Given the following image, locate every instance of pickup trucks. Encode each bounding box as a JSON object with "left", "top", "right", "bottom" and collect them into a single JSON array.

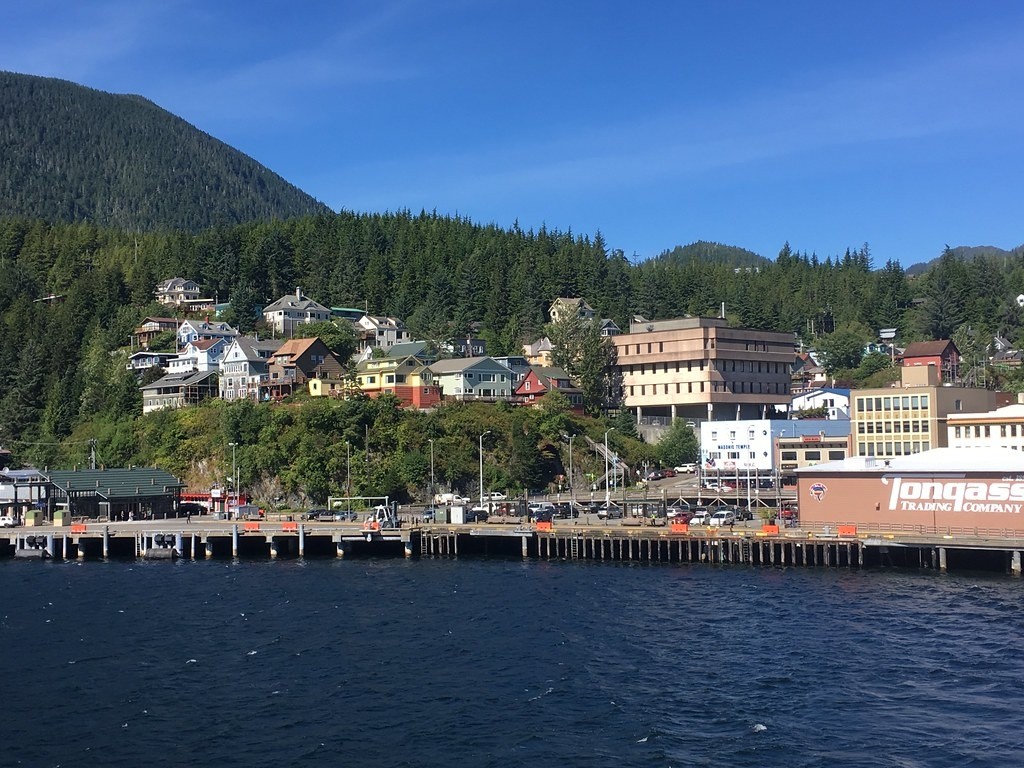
[
  {"left": 553, "top": 505, "right": 579, "bottom": 519},
  {"left": 580, "top": 503, "right": 600, "bottom": 514},
  {"left": 480, "top": 492, "right": 508, "bottom": 501}
]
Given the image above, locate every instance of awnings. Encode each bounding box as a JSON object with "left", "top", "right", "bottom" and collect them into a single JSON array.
[{"left": 778, "top": 500, "right": 798, "bottom": 524}]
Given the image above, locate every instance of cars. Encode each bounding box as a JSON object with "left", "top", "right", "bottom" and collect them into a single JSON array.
[
  {"left": 597, "top": 506, "right": 623, "bottom": 520},
  {"left": 778, "top": 507, "right": 798, "bottom": 520},
  {"left": 177, "top": 502, "right": 207, "bottom": 517},
  {"left": 647, "top": 469, "right": 676, "bottom": 483},
  {"left": 314, "top": 510, "right": 340, "bottom": 521},
  {"left": 674, "top": 464, "right": 698, "bottom": 474},
  {"left": 335, "top": 511, "right": 358, "bottom": 520},
  {"left": 690, "top": 514, "right": 711, "bottom": 526},
  {"left": 300, "top": 509, "right": 325, "bottom": 521},
  {"left": 421, "top": 509, "right": 435, "bottom": 520},
  {"left": 731, "top": 507, "right": 753, "bottom": 520},
  {"left": 632, "top": 504, "right": 733, "bottom": 519},
  {"left": 0, "top": 516, "right": 18, "bottom": 528},
  {"left": 710, "top": 511, "right": 736, "bottom": 527},
  {"left": 651, "top": 420, "right": 661, "bottom": 426},
  {"left": 686, "top": 422, "right": 696, "bottom": 427},
  {"left": 672, "top": 512, "right": 692, "bottom": 525},
  {"left": 706, "top": 483, "right": 732, "bottom": 493},
  {"left": 528, "top": 509, "right": 553, "bottom": 523},
  {"left": 465, "top": 502, "right": 525, "bottom": 522}
]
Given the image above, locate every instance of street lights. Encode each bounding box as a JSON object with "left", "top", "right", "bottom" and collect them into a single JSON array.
[
  {"left": 429, "top": 439, "right": 434, "bottom": 510},
  {"left": 345, "top": 441, "right": 351, "bottom": 521},
  {"left": 479, "top": 431, "right": 491, "bottom": 510},
  {"left": 563, "top": 433, "right": 576, "bottom": 523},
  {"left": 229, "top": 442, "right": 238, "bottom": 508},
  {"left": 604, "top": 427, "right": 615, "bottom": 508}
]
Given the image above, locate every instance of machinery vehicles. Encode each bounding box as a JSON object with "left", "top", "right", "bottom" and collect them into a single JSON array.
[{"left": 363, "top": 500, "right": 401, "bottom": 530}]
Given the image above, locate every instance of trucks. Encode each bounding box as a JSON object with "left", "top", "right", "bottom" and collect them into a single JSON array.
[
  {"left": 435, "top": 493, "right": 463, "bottom": 505},
  {"left": 528, "top": 502, "right": 553, "bottom": 513}
]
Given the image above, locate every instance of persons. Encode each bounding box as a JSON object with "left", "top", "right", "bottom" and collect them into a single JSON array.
[
  {"left": 20, "top": 514, "right": 25, "bottom": 527},
  {"left": 769, "top": 516, "right": 775, "bottom": 525},
  {"left": 650, "top": 513, "right": 656, "bottom": 526},
  {"left": 121, "top": 511, "right": 125, "bottom": 521},
  {"left": 260, "top": 514, "right": 265, "bottom": 518},
  {"left": 143, "top": 511, "right": 147, "bottom": 519},
  {"left": 187, "top": 512, "right": 191, "bottom": 525},
  {"left": 730, "top": 525, "right": 735, "bottom": 532}
]
